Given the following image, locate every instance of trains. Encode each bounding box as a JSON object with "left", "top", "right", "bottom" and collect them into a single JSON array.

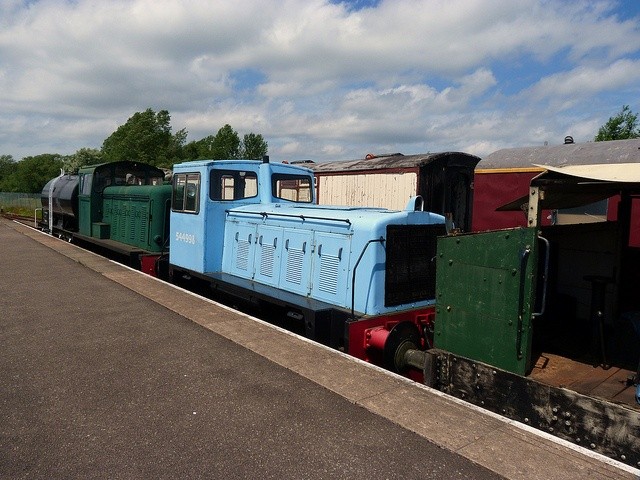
[
  {"left": 125, "top": 135, "right": 640, "bottom": 246},
  {"left": 32, "top": 155, "right": 640, "bottom": 470}
]
